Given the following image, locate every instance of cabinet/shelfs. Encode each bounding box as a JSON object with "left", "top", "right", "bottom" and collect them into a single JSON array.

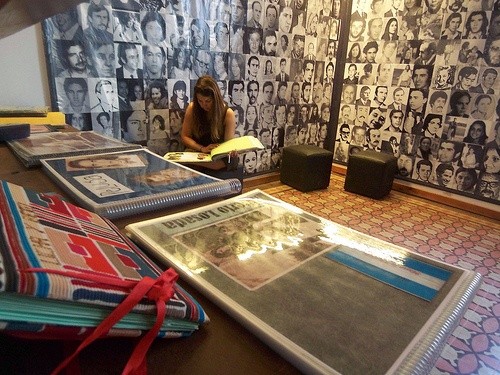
[{"left": 0, "top": 0, "right": 302, "bottom": 375}]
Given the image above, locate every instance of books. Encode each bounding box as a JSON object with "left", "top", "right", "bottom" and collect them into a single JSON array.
[
  {"left": 124, "top": 188, "right": 482, "bottom": 375},
  {"left": 0, "top": 110, "right": 143, "bottom": 169},
  {"left": 162, "top": 135, "right": 265, "bottom": 165},
  {"left": 39, "top": 148, "right": 243, "bottom": 220}
]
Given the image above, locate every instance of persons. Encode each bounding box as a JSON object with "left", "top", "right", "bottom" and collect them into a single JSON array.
[
  {"left": 43, "top": 0, "right": 342, "bottom": 177},
  {"left": 68, "top": 155, "right": 141, "bottom": 169},
  {"left": 176, "top": 76, "right": 240, "bottom": 171},
  {"left": 333, "top": 0, "right": 500, "bottom": 202},
  {"left": 212, "top": 211, "right": 305, "bottom": 258}
]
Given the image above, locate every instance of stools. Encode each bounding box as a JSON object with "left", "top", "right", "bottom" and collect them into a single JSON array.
[
  {"left": 279, "top": 144, "right": 333, "bottom": 192},
  {"left": 343, "top": 149, "right": 399, "bottom": 201}
]
